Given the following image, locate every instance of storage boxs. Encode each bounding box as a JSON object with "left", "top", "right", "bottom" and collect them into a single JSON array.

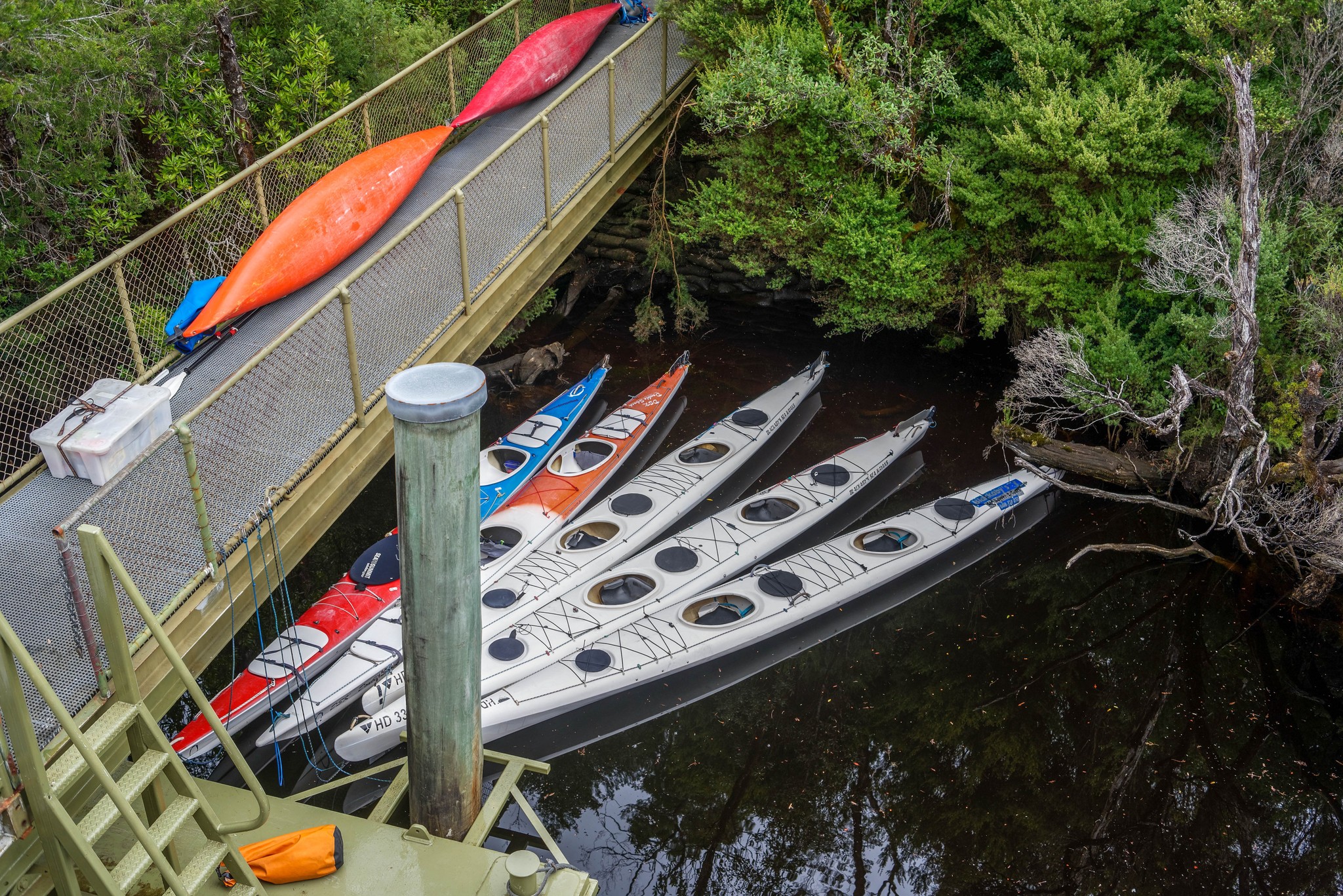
[{"left": 29, "top": 378, "right": 173, "bottom": 487}]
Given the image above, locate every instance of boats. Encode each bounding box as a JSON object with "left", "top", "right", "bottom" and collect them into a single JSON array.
[
  {"left": 483, "top": 493, "right": 1057, "bottom": 783},
  {"left": 342, "top": 453, "right": 927, "bottom": 819},
  {"left": 169, "top": 351, "right": 612, "bottom": 762},
  {"left": 210, "top": 397, "right": 688, "bottom": 788},
  {"left": 405, "top": 461, "right": 1064, "bottom": 761},
  {"left": 450, "top": 0, "right": 627, "bottom": 155},
  {"left": 254, "top": 351, "right": 694, "bottom": 749},
  {"left": 164, "top": 126, "right": 452, "bottom": 355},
  {"left": 328, "top": 403, "right": 941, "bottom": 766},
  {"left": 290, "top": 392, "right": 828, "bottom": 804},
  {"left": 355, "top": 351, "right": 834, "bottom": 718}
]
